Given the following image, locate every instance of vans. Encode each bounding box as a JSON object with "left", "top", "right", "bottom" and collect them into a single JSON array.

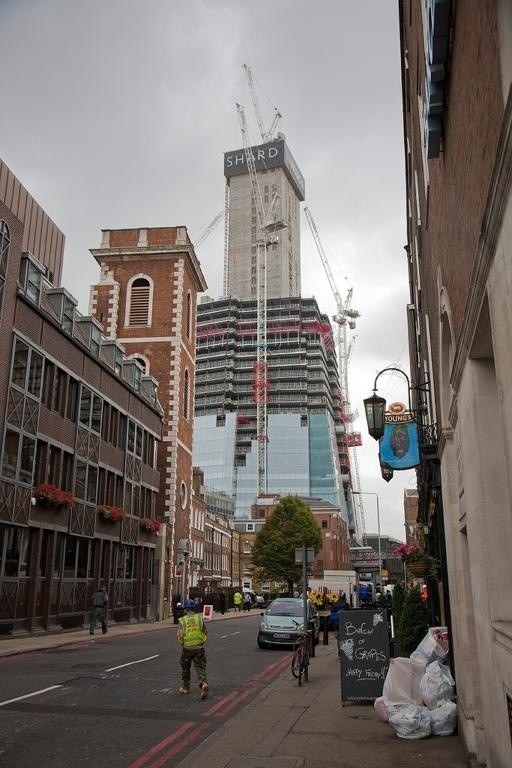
[{"left": 256, "top": 597, "right": 321, "bottom": 650}]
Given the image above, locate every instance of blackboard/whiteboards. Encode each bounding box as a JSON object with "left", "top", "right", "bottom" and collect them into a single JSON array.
[{"left": 338, "top": 610, "right": 390, "bottom": 702}]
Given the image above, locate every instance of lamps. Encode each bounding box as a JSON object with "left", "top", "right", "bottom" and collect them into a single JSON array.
[
  {"left": 381, "top": 462, "right": 395, "bottom": 483},
  {"left": 365, "top": 365, "right": 446, "bottom": 447}
]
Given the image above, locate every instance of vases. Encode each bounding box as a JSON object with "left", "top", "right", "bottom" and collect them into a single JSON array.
[
  {"left": 97, "top": 511, "right": 113, "bottom": 524},
  {"left": 406, "top": 560, "right": 428, "bottom": 577},
  {"left": 140, "top": 525, "right": 150, "bottom": 534},
  {"left": 38, "top": 496, "right": 67, "bottom": 512}
]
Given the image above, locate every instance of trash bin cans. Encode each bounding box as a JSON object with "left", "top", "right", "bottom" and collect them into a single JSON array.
[{"left": 173, "top": 606, "right": 184, "bottom": 624}]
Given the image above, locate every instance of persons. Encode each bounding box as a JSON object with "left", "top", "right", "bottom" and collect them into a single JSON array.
[
  {"left": 176, "top": 598, "right": 209, "bottom": 700},
  {"left": 339, "top": 588, "right": 347, "bottom": 601},
  {"left": 233, "top": 590, "right": 265, "bottom": 613},
  {"left": 330, "top": 597, "right": 350, "bottom": 658},
  {"left": 219, "top": 590, "right": 225, "bottom": 617},
  {"left": 375, "top": 587, "right": 394, "bottom": 610},
  {"left": 88, "top": 585, "right": 108, "bottom": 636},
  {"left": 293, "top": 588, "right": 304, "bottom": 598}
]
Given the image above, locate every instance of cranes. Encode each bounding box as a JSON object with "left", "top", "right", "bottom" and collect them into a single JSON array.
[
  {"left": 234, "top": 102, "right": 290, "bottom": 496},
  {"left": 302, "top": 205, "right": 366, "bottom": 542},
  {"left": 189, "top": 209, "right": 226, "bottom": 252},
  {"left": 241, "top": 61, "right": 282, "bottom": 142}
]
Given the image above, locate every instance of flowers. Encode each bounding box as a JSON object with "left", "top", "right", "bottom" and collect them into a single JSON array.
[
  {"left": 32, "top": 482, "right": 74, "bottom": 507},
  {"left": 96, "top": 502, "right": 125, "bottom": 522},
  {"left": 139, "top": 516, "right": 161, "bottom": 534},
  {"left": 392, "top": 542, "right": 427, "bottom": 562}
]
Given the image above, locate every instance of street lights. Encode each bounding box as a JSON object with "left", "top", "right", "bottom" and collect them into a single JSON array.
[{"left": 351, "top": 491, "right": 383, "bottom": 595}]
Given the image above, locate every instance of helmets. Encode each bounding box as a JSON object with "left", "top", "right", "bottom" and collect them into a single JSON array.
[{"left": 183, "top": 599, "right": 196, "bottom": 608}]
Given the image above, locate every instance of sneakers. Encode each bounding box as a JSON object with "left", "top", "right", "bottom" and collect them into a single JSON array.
[
  {"left": 179, "top": 687, "right": 188, "bottom": 693},
  {"left": 201, "top": 683, "right": 209, "bottom": 699}
]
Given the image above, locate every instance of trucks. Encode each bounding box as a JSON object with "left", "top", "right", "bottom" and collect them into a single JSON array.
[{"left": 243, "top": 587, "right": 265, "bottom": 606}]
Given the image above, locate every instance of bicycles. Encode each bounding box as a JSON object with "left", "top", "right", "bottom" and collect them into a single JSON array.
[{"left": 289, "top": 619, "right": 313, "bottom": 687}]
